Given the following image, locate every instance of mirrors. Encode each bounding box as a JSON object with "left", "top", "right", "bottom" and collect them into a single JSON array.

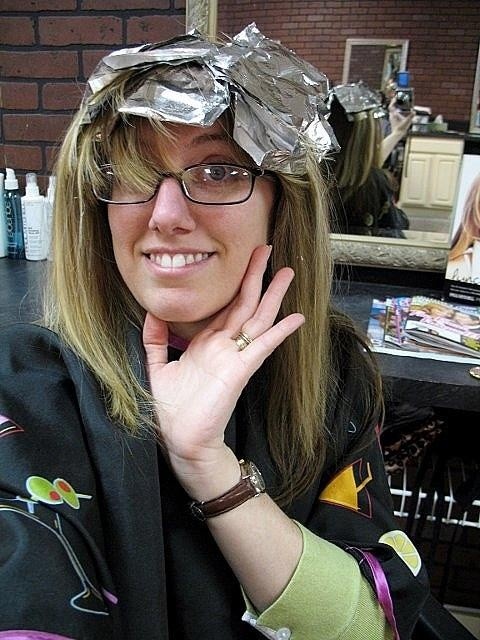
[
  {"left": 186, "top": 0, "right": 480, "bottom": 275},
  {"left": 342, "top": 38, "right": 410, "bottom": 97},
  {"left": 468, "top": 43, "right": 480, "bottom": 134},
  {"left": 381, "top": 47, "right": 403, "bottom": 94}
]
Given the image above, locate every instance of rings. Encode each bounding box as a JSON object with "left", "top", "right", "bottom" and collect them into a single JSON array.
[{"left": 231, "top": 332, "right": 253, "bottom": 351}]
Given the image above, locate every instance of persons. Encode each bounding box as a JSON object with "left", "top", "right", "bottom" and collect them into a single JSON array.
[
  {"left": 0, "top": 21, "right": 433, "bottom": 639},
  {"left": 323, "top": 81, "right": 417, "bottom": 230}
]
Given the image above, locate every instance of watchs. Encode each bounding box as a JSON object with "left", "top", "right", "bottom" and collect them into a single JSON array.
[{"left": 187, "top": 459, "right": 267, "bottom": 523}]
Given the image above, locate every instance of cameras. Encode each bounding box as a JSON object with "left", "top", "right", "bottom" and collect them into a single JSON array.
[{"left": 396, "top": 87, "right": 415, "bottom": 114}]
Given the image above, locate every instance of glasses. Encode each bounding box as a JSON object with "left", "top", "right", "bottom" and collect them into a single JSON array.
[{"left": 86, "top": 158, "right": 276, "bottom": 209}]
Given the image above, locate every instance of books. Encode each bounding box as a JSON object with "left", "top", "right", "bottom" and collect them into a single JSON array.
[
  {"left": 441, "top": 154, "right": 480, "bottom": 305},
  {"left": 361, "top": 293, "right": 480, "bottom": 368}
]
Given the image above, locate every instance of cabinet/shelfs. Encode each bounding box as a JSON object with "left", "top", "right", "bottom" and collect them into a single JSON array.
[{"left": 400, "top": 136, "right": 465, "bottom": 209}]
